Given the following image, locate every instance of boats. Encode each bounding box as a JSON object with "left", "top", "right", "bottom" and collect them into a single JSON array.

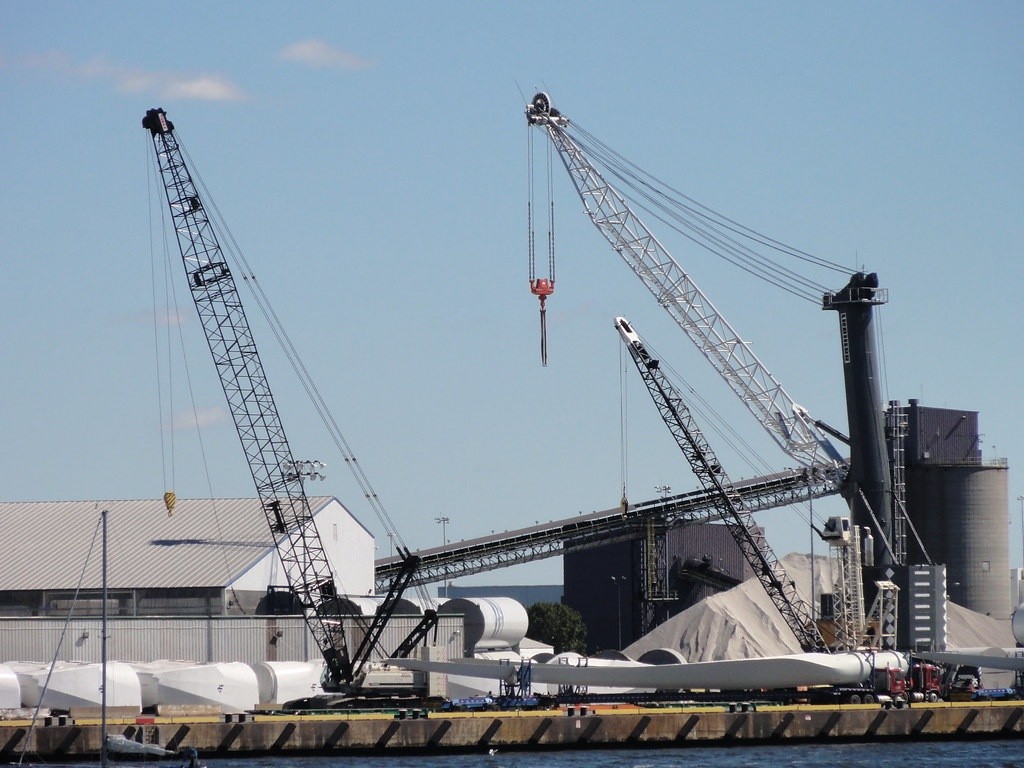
[{"left": 105, "top": 732, "right": 177, "bottom": 761}]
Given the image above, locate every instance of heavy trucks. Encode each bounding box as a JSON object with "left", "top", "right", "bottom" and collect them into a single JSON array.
[{"left": 811, "top": 662, "right": 943, "bottom": 704}]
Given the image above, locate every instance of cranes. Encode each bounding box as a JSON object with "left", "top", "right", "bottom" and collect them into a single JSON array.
[
  {"left": 521, "top": 90, "right": 949, "bottom": 704},
  {"left": 140, "top": 105, "right": 454, "bottom": 714}
]
